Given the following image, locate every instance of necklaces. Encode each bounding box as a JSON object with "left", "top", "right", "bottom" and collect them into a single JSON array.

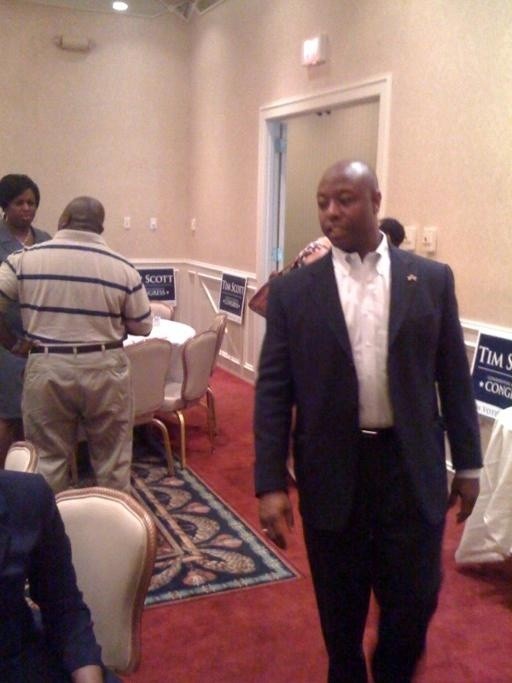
[{"left": 22, "top": 228, "right": 32, "bottom": 246}]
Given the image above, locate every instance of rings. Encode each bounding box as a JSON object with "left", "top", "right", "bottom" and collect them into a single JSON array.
[{"left": 262, "top": 529, "right": 268, "bottom": 533}]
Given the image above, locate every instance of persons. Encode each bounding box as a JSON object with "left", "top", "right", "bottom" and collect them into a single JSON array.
[
  {"left": 251, "top": 159, "right": 484, "bottom": 683},
  {"left": 0, "top": 471, "right": 125, "bottom": 682},
  {"left": 0, "top": 197, "right": 153, "bottom": 498},
  {"left": 0, "top": 174, "right": 53, "bottom": 470},
  {"left": 379, "top": 219, "right": 405, "bottom": 248}
]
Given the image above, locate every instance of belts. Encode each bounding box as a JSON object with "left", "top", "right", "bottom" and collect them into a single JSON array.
[
  {"left": 30, "top": 342, "right": 123, "bottom": 354},
  {"left": 360, "top": 425, "right": 396, "bottom": 445}
]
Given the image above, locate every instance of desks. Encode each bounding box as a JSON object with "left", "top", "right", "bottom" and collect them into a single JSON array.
[{"left": 126, "top": 321, "right": 194, "bottom": 361}]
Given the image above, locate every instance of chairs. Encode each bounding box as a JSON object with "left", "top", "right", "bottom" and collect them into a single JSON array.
[
  {"left": 123, "top": 338, "right": 179, "bottom": 481},
  {"left": 55, "top": 486, "right": 159, "bottom": 676},
  {"left": 148, "top": 300, "right": 177, "bottom": 321},
  {"left": 160, "top": 328, "right": 218, "bottom": 469},
  {"left": 1, "top": 442, "right": 35, "bottom": 476}
]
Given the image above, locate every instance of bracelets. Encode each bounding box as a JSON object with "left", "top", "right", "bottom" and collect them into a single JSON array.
[{"left": 10, "top": 339, "right": 22, "bottom": 354}]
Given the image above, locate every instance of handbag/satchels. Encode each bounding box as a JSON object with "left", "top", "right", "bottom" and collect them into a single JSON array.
[{"left": 248, "top": 257, "right": 297, "bottom": 318}]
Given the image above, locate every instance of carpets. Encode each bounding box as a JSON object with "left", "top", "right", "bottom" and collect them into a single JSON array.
[{"left": 77, "top": 435, "right": 303, "bottom": 608}]
